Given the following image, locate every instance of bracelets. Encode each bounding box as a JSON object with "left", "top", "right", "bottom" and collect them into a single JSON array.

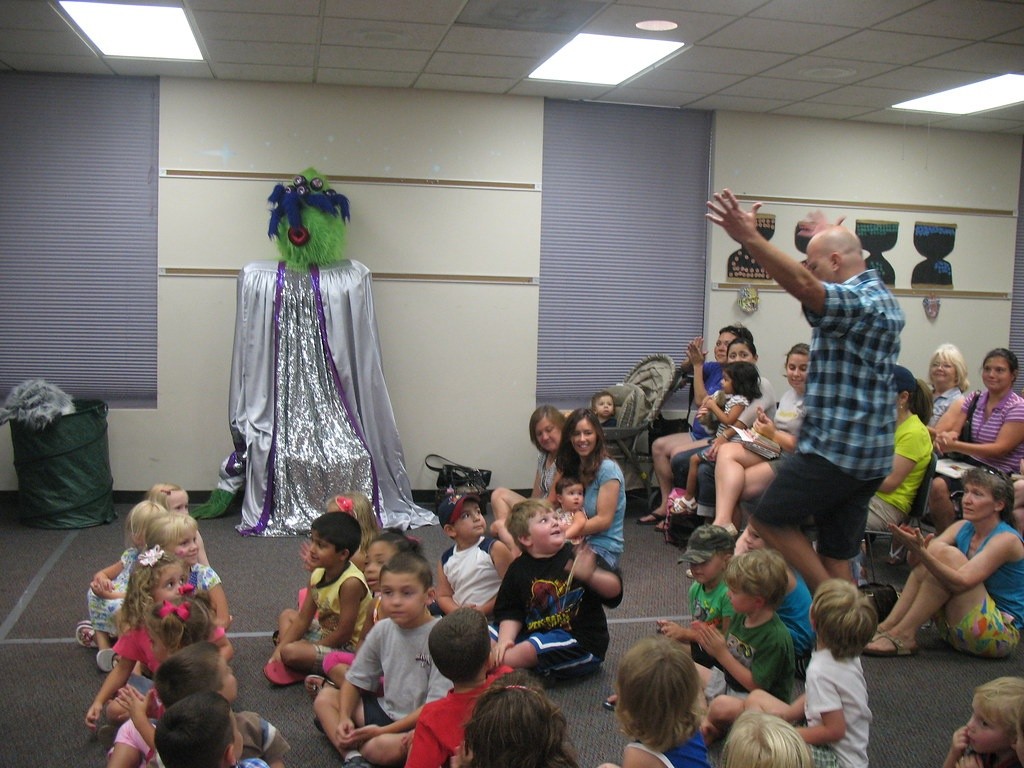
[{"left": 722, "top": 432, "right": 730, "bottom": 441}]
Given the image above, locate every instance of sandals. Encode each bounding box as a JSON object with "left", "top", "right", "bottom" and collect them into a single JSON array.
[
  {"left": 654, "top": 520, "right": 670, "bottom": 533},
  {"left": 76, "top": 624, "right": 103, "bottom": 649},
  {"left": 672, "top": 496, "right": 697, "bottom": 514},
  {"left": 635, "top": 511, "right": 667, "bottom": 524},
  {"left": 303, "top": 673, "right": 338, "bottom": 693},
  {"left": 96, "top": 647, "right": 118, "bottom": 673},
  {"left": 888, "top": 540, "right": 908, "bottom": 566}
]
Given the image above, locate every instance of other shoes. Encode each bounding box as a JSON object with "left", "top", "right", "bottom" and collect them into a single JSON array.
[
  {"left": 339, "top": 756, "right": 377, "bottom": 767},
  {"left": 264, "top": 658, "right": 305, "bottom": 688},
  {"left": 723, "top": 523, "right": 740, "bottom": 536}
]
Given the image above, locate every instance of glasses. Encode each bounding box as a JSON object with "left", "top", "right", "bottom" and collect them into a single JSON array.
[{"left": 980, "top": 464, "right": 1002, "bottom": 478}]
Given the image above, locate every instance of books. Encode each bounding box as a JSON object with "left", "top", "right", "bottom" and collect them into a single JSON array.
[{"left": 742, "top": 427, "right": 781, "bottom": 459}]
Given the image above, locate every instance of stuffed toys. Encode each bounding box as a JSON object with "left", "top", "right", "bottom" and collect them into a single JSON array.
[{"left": 190, "top": 165, "right": 440, "bottom": 538}]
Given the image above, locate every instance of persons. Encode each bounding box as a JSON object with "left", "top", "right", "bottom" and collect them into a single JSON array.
[
  {"left": 488, "top": 498, "right": 623, "bottom": 677},
  {"left": 926, "top": 342, "right": 1024, "bottom": 543},
  {"left": 637, "top": 325, "right": 777, "bottom": 547},
  {"left": 863, "top": 468, "right": 1024, "bottom": 657},
  {"left": 450, "top": 669, "right": 580, "bottom": 768},
  {"left": 598, "top": 634, "right": 712, "bottom": 768},
  {"left": 744, "top": 577, "right": 878, "bottom": 768},
  {"left": 427, "top": 494, "right": 513, "bottom": 621},
  {"left": 263, "top": 492, "right": 423, "bottom": 697},
  {"left": 943, "top": 677, "right": 1024, "bottom": 768},
  {"left": 564, "top": 391, "right": 617, "bottom": 427},
  {"left": 75, "top": 483, "right": 291, "bottom": 768},
  {"left": 490, "top": 404, "right": 626, "bottom": 570},
  {"left": 602, "top": 521, "right": 815, "bottom": 748},
  {"left": 718, "top": 712, "right": 816, "bottom": 768},
  {"left": 864, "top": 365, "right": 934, "bottom": 531},
  {"left": 404, "top": 606, "right": 514, "bottom": 768},
  {"left": 705, "top": 189, "right": 906, "bottom": 600},
  {"left": 686, "top": 344, "right": 811, "bottom": 578},
  {"left": 313, "top": 552, "right": 454, "bottom": 768}
]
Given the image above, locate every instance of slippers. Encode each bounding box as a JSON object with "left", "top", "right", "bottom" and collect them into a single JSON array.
[{"left": 864, "top": 627, "right": 919, "bottom": 656}]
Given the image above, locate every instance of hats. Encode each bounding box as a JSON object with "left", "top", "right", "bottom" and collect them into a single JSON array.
[
  {"left": 437, "top": 491, "right": 483, "bottom": 527},
  {"left": 677, "top": 524, "right": 735, "bottom": 559}
]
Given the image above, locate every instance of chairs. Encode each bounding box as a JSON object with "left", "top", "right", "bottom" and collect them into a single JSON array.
[{"left": 866, "top": 454, "right": 943, "bottom": 586}]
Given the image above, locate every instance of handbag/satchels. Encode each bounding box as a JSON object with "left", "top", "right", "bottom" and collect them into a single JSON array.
[
  {"left": 649, "top": 416, "right": 693, "bottom": 451},
  {"left": 424, "top": 453, "right": 493, "bottom": 516},
  {"left": 862, "top": 581, "right": 899, "bottom": 624},
  {"left": 948, "top": 389, "right": 983, "bottom": 459}
]
